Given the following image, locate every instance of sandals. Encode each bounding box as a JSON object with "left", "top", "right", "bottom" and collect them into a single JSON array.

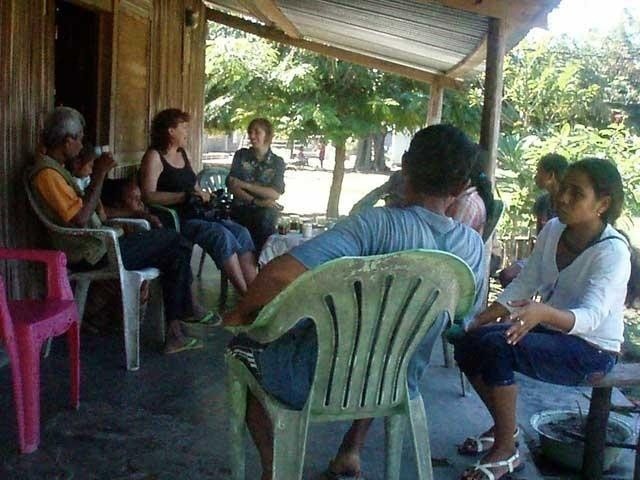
[
  {"left": 456, "top": 447, "right": 525, "bottom": 480},
  {"left": 457, "top": 425, "right": 520, "bottom": 454}
]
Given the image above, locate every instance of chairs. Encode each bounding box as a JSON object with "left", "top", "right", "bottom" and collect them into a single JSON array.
[{"left": 225, "top": 249, "right": 476, "bottom": 479}]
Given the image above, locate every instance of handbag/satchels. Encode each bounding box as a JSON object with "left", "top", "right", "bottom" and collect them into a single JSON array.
[{"left": 180, "top": 189, "right": 227, "bottom": 221}]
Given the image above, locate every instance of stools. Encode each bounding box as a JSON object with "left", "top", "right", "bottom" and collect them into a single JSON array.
[{"left": 568, "top": 363, "right": 640, "bottom": 479}]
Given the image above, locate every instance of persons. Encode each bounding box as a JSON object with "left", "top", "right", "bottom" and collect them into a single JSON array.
[
  {"left": 139, "top": 107, "right": 257, "bottom": 292},
  {"left": 436, "top": 144, "right": 494, "bottom": 240},
  {"left": 226, "top": 119, "right": 287, "bottom": 259},
  {"left": 222, "top": 122, "right": 490, "bottom": 480},
  {"left": 496, "top": 150, "right": 569, "bottom": 287},
  {"left": 28, "top": 103, "right": 223, "bottom": 358},
  {"left": 349, "top": 169, "right": 407, "bottom": 217},
  {"left": 453, "top": 156, "right": 640, "bottom": 480}
]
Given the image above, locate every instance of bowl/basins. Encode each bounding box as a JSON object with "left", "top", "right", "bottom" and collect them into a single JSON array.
[{"left": 530, "top": 408, "right": 635, "bottom": 473}]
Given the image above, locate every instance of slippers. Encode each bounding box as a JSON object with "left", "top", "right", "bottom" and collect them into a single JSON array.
[
  {"left": 163, "top": 336, "right": 206, "bottom": 353},
  {"left": 178, "top": 310, "right": 223, "bottom": 328}
]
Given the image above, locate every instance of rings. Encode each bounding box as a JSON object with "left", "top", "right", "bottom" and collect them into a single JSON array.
[{"left": 517, "top": 318, "right": 525, "bottom": 327}]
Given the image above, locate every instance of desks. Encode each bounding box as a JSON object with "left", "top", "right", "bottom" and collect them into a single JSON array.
[{"left": 261, "top": 230, "right": 320, "bottom": 263}]
